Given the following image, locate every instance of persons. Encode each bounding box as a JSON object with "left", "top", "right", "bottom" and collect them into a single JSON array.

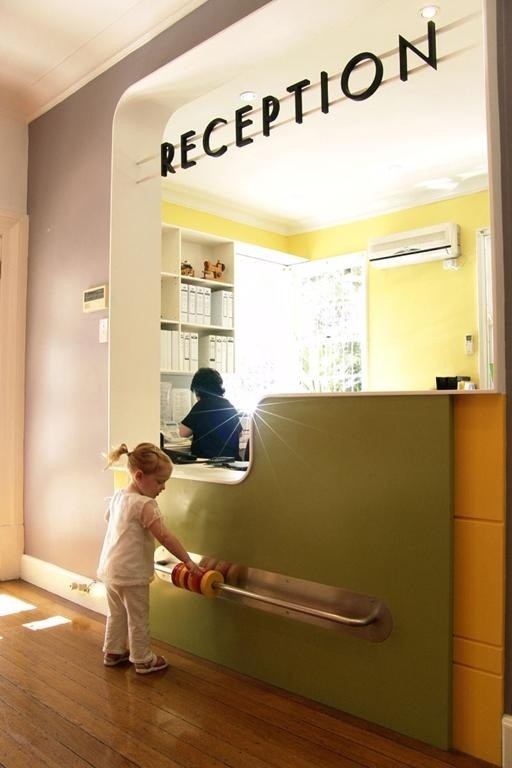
[
  {"left": 96, "top": 441, "right": 208, "bottom": 673},
  {"left": 178, "top": 367, "right": 244, "bottom": 463}
]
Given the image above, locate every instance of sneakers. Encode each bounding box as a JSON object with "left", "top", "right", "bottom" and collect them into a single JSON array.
[
  {"left": 134, "top": 653, "right": 168, "bottom": 674},
  {"left": 103, "top": 648, "right": 131, "bottom": 666}
]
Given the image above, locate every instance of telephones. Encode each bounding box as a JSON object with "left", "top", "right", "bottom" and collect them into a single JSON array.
[{"left": 464, "top": 334, "right": 475, "bottom": 356}]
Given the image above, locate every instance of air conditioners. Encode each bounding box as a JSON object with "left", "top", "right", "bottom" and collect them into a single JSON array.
[{"left": 366, "top": 223, "right": 462, "bottom": 270}]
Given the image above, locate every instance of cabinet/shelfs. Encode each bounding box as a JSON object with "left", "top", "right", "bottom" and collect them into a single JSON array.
[{"left": 162, "top": 223, "right": 237, "bottom": 430}]
